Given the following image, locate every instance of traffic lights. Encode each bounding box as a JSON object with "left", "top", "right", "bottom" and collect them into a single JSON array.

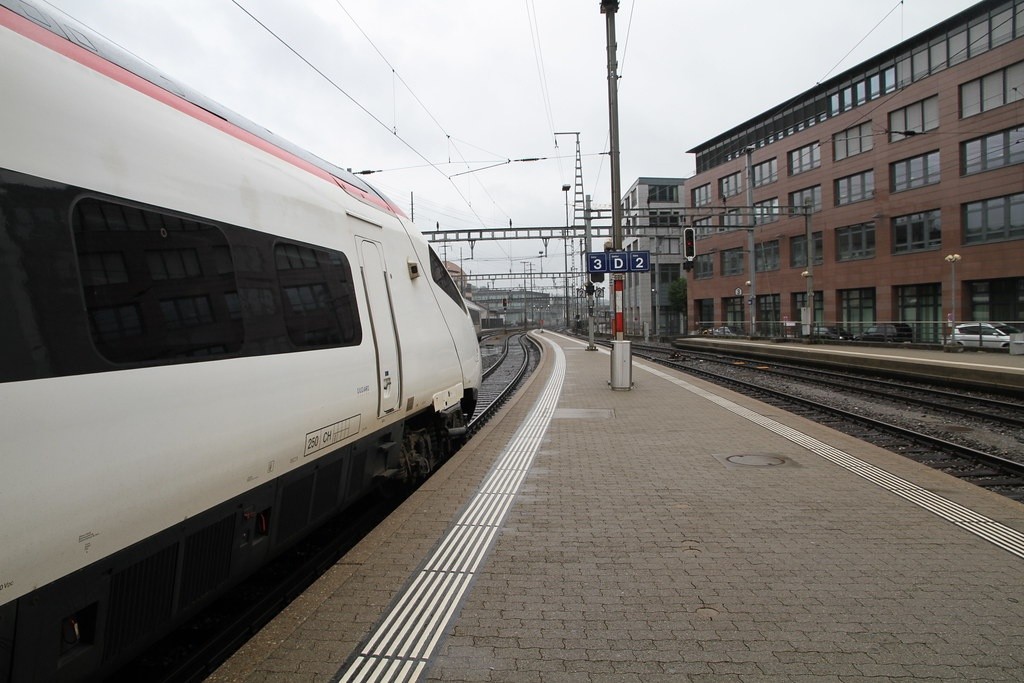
[
  {"left": 684, "top": 228, "right": 696, "bottom": 262},
  {"left": 503, "top": 299, "right": 507, "bottom": 307}
]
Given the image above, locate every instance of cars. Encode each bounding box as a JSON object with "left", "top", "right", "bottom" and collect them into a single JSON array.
[
  {"left": 951, "top": 321, "right": 1021, "bottom": 349},
  {"left": 714, "top": 325, "right": 747, "bottom": 336},
  {"left": 812, "top": 325, "right": 854, "bottom": 340},
  {"left": 854, "top": 322, "right": 913, "bottom": 343}
]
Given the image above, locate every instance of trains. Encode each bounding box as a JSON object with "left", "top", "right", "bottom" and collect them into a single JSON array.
[{"left": 1, "top": 1, "right": 481, "bottom": 682}]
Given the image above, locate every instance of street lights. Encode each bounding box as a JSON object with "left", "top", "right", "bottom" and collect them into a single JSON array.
[
  {"left": 562, "top": 183, "right": 571, "bottom": 235},
  {"left": 945, "top": 253, "right": 962, "bottom": 343},
  {"left": 744, "top": 145, "right": 757, "bottom": 339}
]
